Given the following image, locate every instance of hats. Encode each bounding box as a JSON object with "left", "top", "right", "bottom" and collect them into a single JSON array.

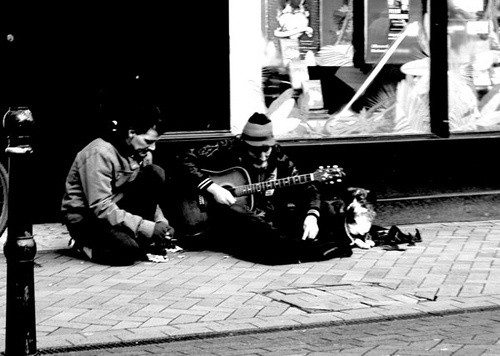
[{"left": 242, "top": 112, "right": 277, "bottom": 146}]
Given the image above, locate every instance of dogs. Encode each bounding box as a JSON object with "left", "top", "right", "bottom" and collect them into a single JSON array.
[{"left": 323, "top": 181, "right": 378, "bottom": 250}]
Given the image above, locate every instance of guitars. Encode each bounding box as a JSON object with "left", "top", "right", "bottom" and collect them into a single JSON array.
[{"left": 174, "top": 162, "right": 344, "bottom": 239}]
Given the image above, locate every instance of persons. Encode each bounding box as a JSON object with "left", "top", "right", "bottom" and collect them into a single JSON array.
[
  {"left": 61, "top": 103, "right": 176, "bottom": 267},
  {"left": 175, "top": 113, "right": 320, "bottom": 239}
]
